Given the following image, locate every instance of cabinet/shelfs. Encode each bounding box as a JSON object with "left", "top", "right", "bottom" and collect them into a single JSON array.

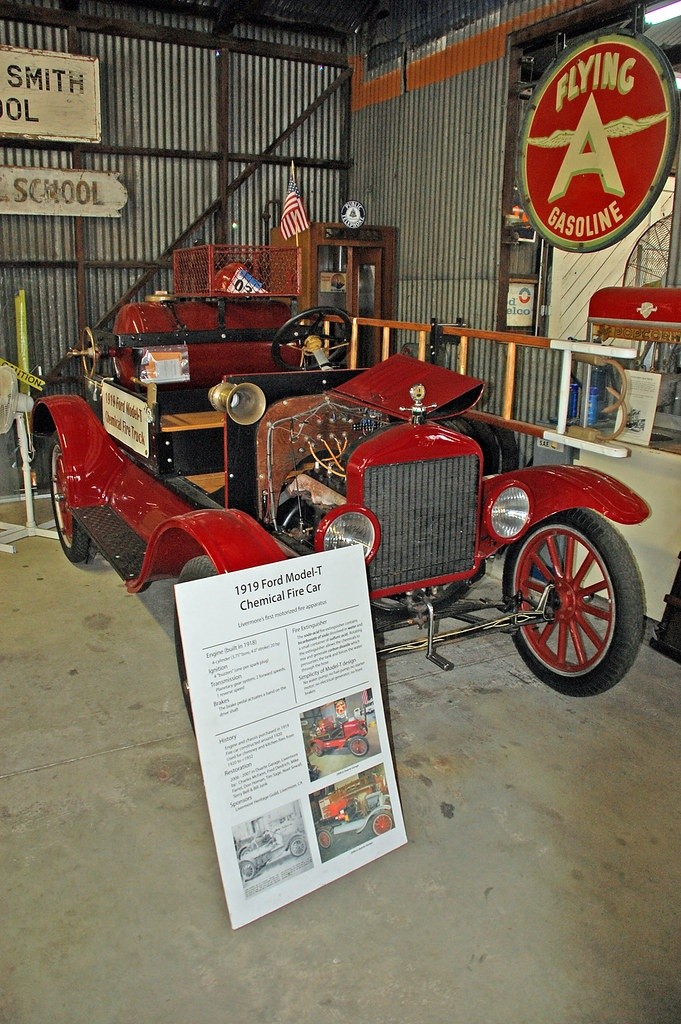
[{"left": 271, "top": 223, "right": 397, "bottom": 369}]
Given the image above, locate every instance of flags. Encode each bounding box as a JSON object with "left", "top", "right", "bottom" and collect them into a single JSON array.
[{"left": 280, "top": 162, "right": 310, "bottom": 240}]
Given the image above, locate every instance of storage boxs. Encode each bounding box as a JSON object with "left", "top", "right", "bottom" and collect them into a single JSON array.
[{"left": 172, "top": 244, "right": 303, "bottom": 297}]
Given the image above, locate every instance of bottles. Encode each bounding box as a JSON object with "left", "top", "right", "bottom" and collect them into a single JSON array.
[{"left": 569, "top": 365, "right": 604, "bottom": 427}]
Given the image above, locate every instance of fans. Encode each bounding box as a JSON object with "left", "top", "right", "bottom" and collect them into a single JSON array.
[{"left": 0, "top": 365, "right": 59, "bottom": 554}]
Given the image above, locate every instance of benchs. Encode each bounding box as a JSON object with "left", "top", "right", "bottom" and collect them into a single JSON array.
[{"left": 129, "top": 380, "right": 225, "bottom": 473}]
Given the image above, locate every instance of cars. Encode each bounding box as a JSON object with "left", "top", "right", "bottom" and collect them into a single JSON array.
[
  {"left": 29, "top": 290, "right": 652, "bottom": 696},
  {"left": 237, "top": 818, "right": 307, "bottom": 881},
  {"left": 315, "top": 777, "right": 392, "bottom": 849},
  {"left": 308, "top": 715, "right": 369, "bottom": 757}
]
